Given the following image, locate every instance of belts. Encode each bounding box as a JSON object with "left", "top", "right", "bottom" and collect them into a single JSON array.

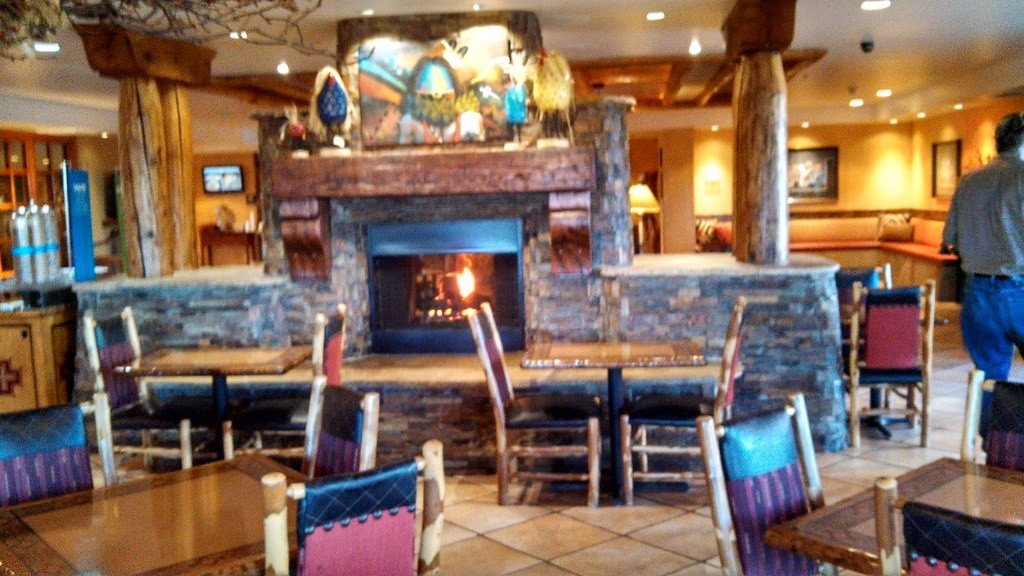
[{"left": 973, "top": 272, "right": 1011, "bottom": 282}]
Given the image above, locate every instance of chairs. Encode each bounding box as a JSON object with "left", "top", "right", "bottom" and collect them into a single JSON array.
[{"left": 0, "top": 263, "right": 1024, "bottom": 576}]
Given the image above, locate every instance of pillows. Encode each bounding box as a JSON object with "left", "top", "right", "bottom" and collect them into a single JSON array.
[
  {"left": 878, "top": 212, "right": 914, "bottom": 241},
  {"left": 695, "top": 217, "right": 733, "bottom": 252}
]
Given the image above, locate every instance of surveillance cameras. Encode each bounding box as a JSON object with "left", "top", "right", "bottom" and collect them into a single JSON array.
[{"left": 861, "top": 41, "right": 874, "bottom": 52}]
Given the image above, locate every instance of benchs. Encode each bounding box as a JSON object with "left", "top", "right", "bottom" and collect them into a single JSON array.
[{"left": 778, "top": 218, "right": 964, "bottom": 301}]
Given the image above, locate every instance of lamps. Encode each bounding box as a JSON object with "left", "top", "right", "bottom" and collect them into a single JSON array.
[{"left": 628, "top": 184, "right": 660, "bottom": 243}]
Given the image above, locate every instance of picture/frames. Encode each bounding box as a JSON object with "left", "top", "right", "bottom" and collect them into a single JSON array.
[
  {"left": 787, "top": 146, "right": 839, "bottom": 202},
  {"left": 930, "top": 139, "right": 962, "bottom": 197}
]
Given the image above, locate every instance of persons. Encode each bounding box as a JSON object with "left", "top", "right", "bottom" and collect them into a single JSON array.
[{"left": 942, "top": 113, "right": 1024, "bottom": 452}]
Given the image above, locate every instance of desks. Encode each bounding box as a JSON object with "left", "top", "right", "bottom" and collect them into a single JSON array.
[
  {"left": 520, "top": 340, "right": 707, "bottom": 507},
  {"left": 765, "top": 458, "right": 1024, "bottom": 576},
  {"left": 117, "top": 345, "right": 313, "bottom": 461},
  {"left": 0, "top": 449, "right": 316, "bottom": 575},
  {"left": 838, "top": 305, "right": 948, "bottom": 439},
  {"left": 200, "top": 225, "right": 261, "bottom": 266}
]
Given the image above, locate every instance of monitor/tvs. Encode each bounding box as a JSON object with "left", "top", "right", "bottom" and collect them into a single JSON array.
[{"left": 202, "top": 164, "right": 244, "bottom": 193}]
